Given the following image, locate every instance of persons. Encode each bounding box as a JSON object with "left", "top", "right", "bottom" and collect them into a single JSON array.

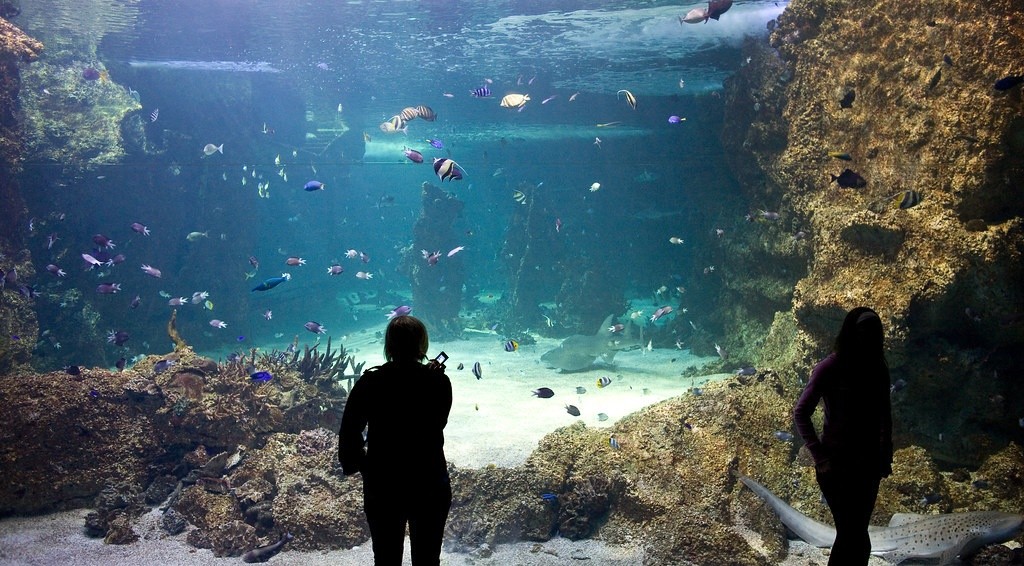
[
  {"left": 338, "top": 314, "right": 454, "bottom": 566},
  {"left": 794, "top": 307, "right": 895, "bottom": 566}
]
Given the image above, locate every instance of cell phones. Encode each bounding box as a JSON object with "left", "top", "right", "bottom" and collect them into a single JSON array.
[{"left": 432, "top": 351, "right": 449, "bottom": 367}]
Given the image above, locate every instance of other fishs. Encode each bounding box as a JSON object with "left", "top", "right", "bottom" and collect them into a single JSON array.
[{"left": 0, "top": 0, "right": 1024, "bottom": 566}]
